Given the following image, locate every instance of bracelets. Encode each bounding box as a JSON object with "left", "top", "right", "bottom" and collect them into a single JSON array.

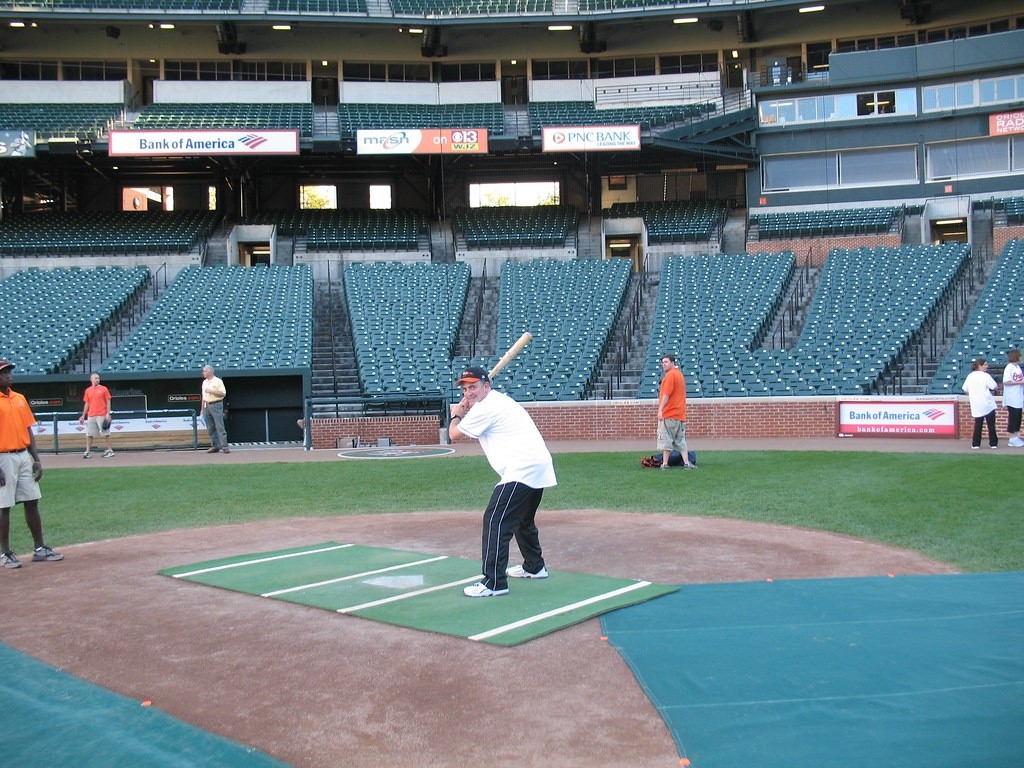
[
  {"left": 450, "top": 415, "right": 461, "bottom": 422},
  {"left": 34, "top": 462, "right": 41, "bottom": 464}
]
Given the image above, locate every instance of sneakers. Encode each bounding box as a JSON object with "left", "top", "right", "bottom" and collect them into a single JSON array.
[
  {"left": 83, "top": 452, "right": 91, "bottom": 458},
  {"left": 1009, "top": 437, "right": 1024, "bottom": 447},
  {"left": 507, "top": 564, "right": 548, "bottom": 578},
  {"left": 684, "top": 462, "right": 697, "bottom": 470},
  {"left": 102, "top": 450, "right": 115, "bottom": 457},
  {"left": 660, "top": 464, "right": 669, "bottom": 470},
  {"left": 1, "top": 551, "right": 23, "bottom": 568},
  {"left": 463, "top": 582, "right": 509, "bottom": 596},
  {"left": 32, "top": 546, "right": 63, "bottom": 561}
]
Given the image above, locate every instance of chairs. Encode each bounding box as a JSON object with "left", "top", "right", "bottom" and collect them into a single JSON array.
[{"left": 0, "top": 0, "right": 1024, "bottom": 396}]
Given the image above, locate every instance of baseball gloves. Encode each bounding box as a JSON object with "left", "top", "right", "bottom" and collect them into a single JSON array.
[{"left": 102, "top": 419, "right": 110, "bottom": 430}]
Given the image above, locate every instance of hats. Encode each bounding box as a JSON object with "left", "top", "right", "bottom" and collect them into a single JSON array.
[
  {"left": 455, "top": 367, "right": 489, "bottom": 387},
  {"left": 0, "top": 360, "right": 15, "bottom": 371}
]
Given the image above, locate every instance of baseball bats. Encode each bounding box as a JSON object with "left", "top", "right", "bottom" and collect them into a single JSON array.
[{"left": 487, "top": 332, "right": 532, "bottom": 381}]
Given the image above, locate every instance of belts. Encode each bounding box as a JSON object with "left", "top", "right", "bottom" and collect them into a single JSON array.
[
  {"left": 2, "top": 449, "right": 26, "bottom": 453},
  {"left": 209, "top": 401, "right": 221, "bottom": 404}
]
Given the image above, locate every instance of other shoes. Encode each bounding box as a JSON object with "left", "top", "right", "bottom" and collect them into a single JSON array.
[
  {"left": 991, "top": 446, "right": 997, "bottom": 449},
  {"left": 971, "top": 446, "right": 979, "bottom": 449},
  {"left": 223, "top": 448, "right": 230, "bottom": 453},
  {"left": 207, "top": 447, "right": 219, "bottom": 453}
]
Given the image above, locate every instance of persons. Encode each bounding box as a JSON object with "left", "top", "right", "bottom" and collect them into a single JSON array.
[
  {"left": 1002, "top": 350, "right": 1024, "bottom": 447},
  {"left": 449, "top": 367, "right": 557, "bottom": 597},
  {"left": 0, "top": 360, "right": 63, "bottom": 567},
  {"left": 962, "top": 359, "right": 999, "bottom": 449},
  {"left": 657, "top": 355, "right": 697, "bottom": 470},
  {"left": 200, "top": 365, "right": 230, "bottom": 453},
  {"left": 79, "top": 374, "right": 115, "bottom": 459}
]
